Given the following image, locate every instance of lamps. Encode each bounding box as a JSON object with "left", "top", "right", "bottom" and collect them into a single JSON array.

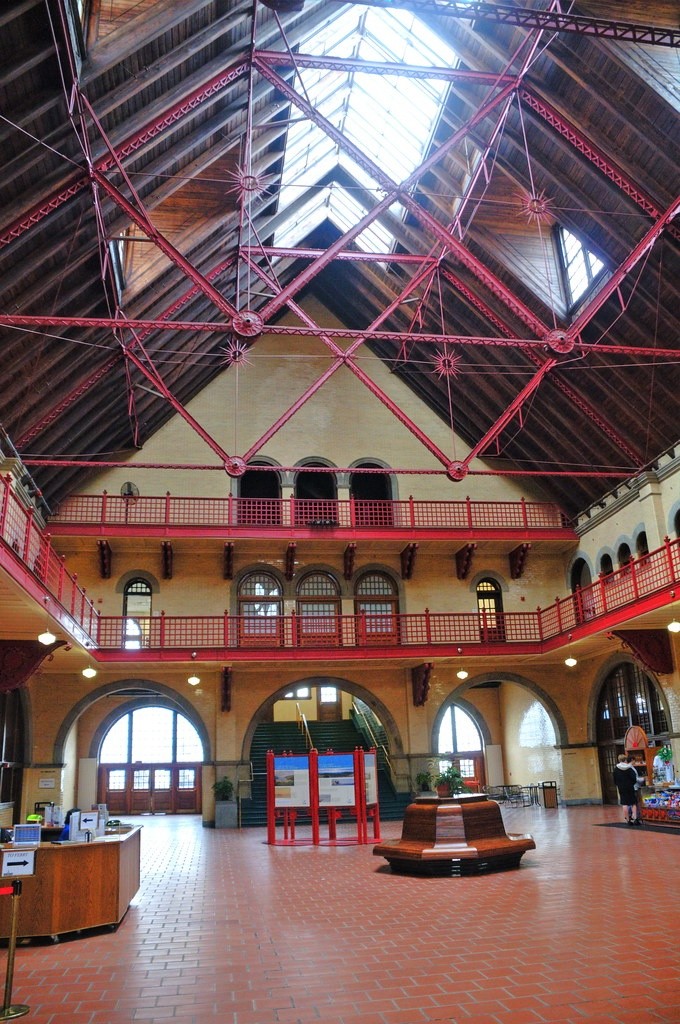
[
  {"left": 82, "top": 645, "right": 96, "bottom": 679},
  {"left": 38, "top": 597, "right": 56, "bottom": 645},
  {"left": 456, "top": 648, "right": 468, "bottom": 679},
  {"left": 565, "top": 634, "right": 577, "bottom": 667},
  {"left": 667, "top": 591, "right": 680, "bottom": 633},
  {"left": 187, "top": 652, "right": 200, "bottom": 686}
]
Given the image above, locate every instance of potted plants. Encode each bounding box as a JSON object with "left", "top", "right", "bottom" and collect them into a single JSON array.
[
  {"left": 435, "top": 767, "right": 463, "bottom": 798},
  {"left": 415, "top": 772, "right": 432, "bottom": 791},
  {"left": 212, "top": 777, "right": 233, "bottom": 801}
]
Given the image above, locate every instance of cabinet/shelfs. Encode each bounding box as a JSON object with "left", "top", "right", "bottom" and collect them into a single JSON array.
[
  {"left": 639, "top": 786, "right": 680, "bottom": 828},
  {"left": 626, "top": 748, "right": 661, "bottom": 785}
]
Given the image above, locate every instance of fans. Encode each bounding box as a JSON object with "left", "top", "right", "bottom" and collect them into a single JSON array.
[{"left": 120, "top": 482, "right": 140, "bottom": 524}]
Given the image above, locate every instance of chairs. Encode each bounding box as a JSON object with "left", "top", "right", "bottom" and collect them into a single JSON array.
[{"left": 483, "top": 783, "right": 540, "bottom": 808}]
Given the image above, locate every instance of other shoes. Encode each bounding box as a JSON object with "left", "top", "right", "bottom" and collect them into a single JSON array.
[
  {"left": 626, "top": 820, "right": 632, "bottom": 825},
  {"left": 634, "top": 819, "right": 640, "bottom": 824}
]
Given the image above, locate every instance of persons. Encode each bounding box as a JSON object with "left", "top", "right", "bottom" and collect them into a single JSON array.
[
  {"left": 613, "top": 754, "right": 647, "bottom": 825},
  {"left": 57, "top": 808, "right": 81, "bottom": 841}
]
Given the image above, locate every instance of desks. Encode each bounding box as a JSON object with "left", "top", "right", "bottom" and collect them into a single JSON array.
[
  {"left": 0, "top": 824, "right": 143, "bottom": 938},
  {"left": 522, "top": 787, "right": 541, "bottom": 807},
  {"left": 498, "top": 785, "right": 519, "bottom": 804}
]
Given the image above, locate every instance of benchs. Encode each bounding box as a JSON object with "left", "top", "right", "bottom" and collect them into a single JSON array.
[{"left": 373, "top": 803, "right": 536, "bottom": 862}]
